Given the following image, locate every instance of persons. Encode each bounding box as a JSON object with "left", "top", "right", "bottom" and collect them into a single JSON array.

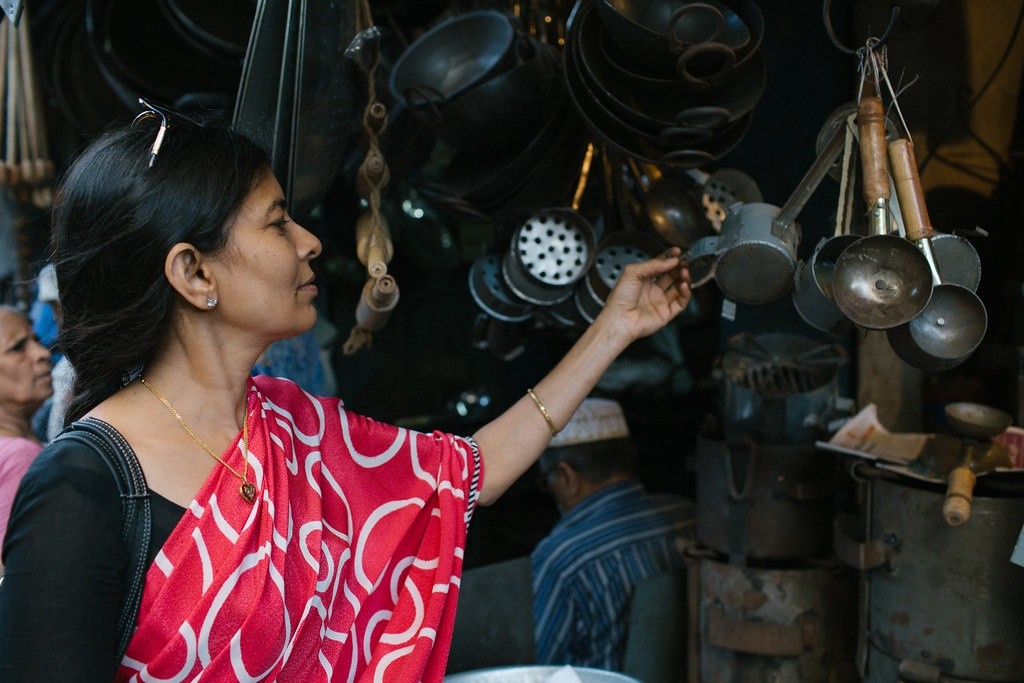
[
  {"left": 1, "top": 117, "right": 691, "bottom": 683},
  {"left": 0, "top": 258, "right": 342, "bottom": 584},
  {"left": 529, "top": 397, "right": 699, "bottom": 673}
]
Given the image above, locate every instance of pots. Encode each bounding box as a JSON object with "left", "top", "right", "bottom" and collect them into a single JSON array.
[
  {"left": 833, "top": 461, "right": 1024, "bottom": 683},
  {"left": 694, "top": 432, "right": 834, "bottom": 559},
  {"left": 389, "top": 0, "right": 766, "bottom": 223}
]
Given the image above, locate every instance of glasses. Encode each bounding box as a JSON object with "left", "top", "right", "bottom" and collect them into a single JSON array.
[{"left": 132, "top": 93, "right": 208, "bottom": 170}]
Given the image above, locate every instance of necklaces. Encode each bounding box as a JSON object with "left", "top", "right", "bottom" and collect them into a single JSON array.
[{"left": 139, "top": 370, "right": 256, "bottom": 504}]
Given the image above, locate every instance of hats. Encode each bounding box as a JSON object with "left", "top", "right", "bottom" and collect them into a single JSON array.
[{"left": 549, "top": 397, "right": 629, "bottom": 446}]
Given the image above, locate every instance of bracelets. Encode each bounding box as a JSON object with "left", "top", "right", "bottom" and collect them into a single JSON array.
[{"left": 528, "top": 388, "right": 558, "bottom": 436}]
